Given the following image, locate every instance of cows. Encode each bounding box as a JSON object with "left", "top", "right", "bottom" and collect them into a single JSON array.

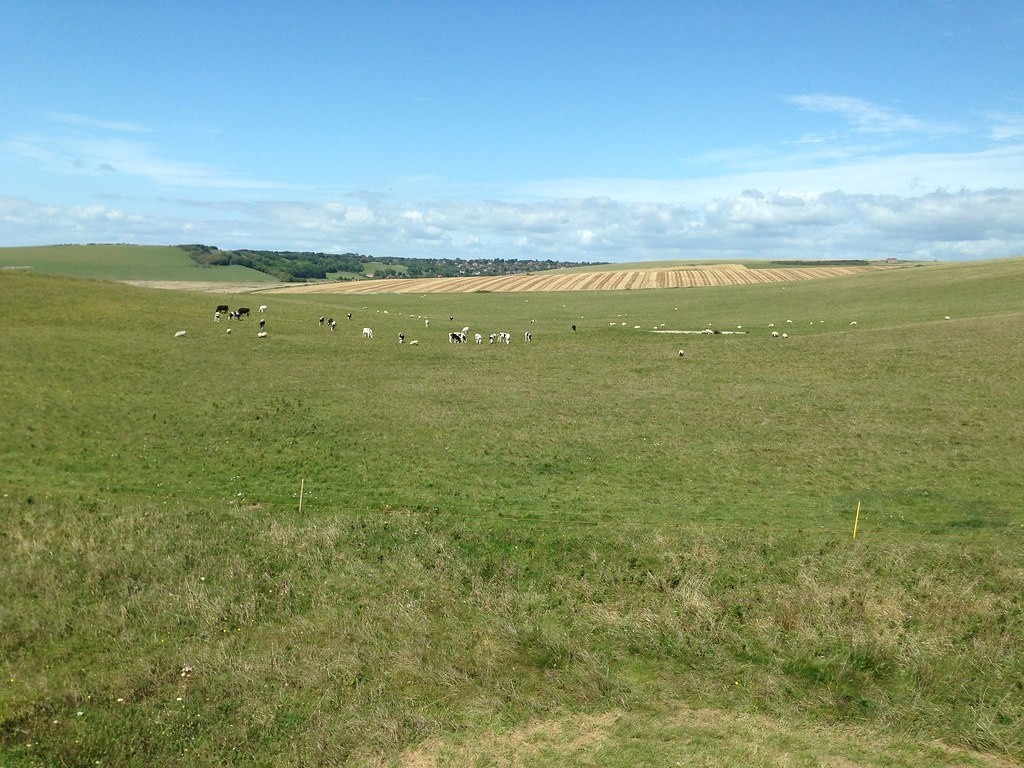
[
  {"left": 347, "top": 312, "right": 351, "bottom": 319},
  {"left": 609, "top": 307, "right": 826, "bottom": 339},
  {"left": 319, "top": 316, "right": 337, "bottom": 331},
  {"left": 174, "top": 330, "right": 187, "bottom": 337},
  {"left": 474, "top": 332, "right": 511, "bottom": 345},
  {"left": 213, "top": 305, "right": 268, "bottom": 337},
  {"left": 572, "top": 323, "right": 578, "bottom": 332},
  {"left": 461, "top": 327, "right": 469, "bottom": 331},
  {"left": 524, "top": 331, "right": 532, "bottom": 341},
  {"left": 362, "top": 327, "right": 374, "bottom": 339},
  {"left": 849, "top": 322, "right": 858, "bottom": 325},
  {"left": 449, "top": 331, "right": 467, "bottom": 346},
  {"left": 678, "top": 349, "right": 685, "bottom": 357},
  {"left": 361, "top": 305, "right": 430, "bottom": 329},
  {"left": 945, "top": 316, "right": 950, "bottom": 320},
  {"left": 531, "top": 319, "right": 537, "bottom": 325}
]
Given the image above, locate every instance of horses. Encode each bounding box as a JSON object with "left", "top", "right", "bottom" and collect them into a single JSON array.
[{"left": 397, "top": 331, "right": 405, "bottom": 344}]
